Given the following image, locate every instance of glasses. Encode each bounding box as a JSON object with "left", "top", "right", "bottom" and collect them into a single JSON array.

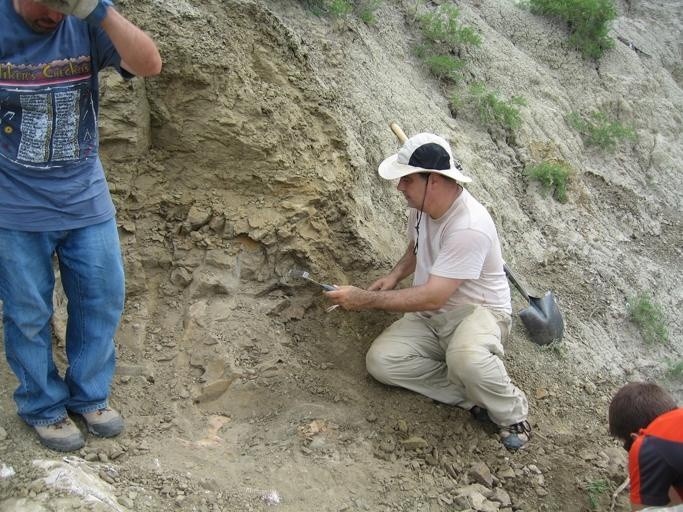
[{"left": 623, "top": 436, "right": 634, "bottom": 452}]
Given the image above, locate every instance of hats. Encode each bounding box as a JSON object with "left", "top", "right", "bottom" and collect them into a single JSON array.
[{"left": 377, "top": 132, "right": 472, "bottom": 183}]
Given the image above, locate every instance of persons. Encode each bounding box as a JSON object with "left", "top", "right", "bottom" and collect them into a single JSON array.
[
  {"left": 607, "top": 381, "right": 683, "bottom": 512},
  {"left": 322, "top": 134, "right": 532, "bottom": 451},
  {"left": 0, "top": 0, "right": 162, "bottom": 450}
]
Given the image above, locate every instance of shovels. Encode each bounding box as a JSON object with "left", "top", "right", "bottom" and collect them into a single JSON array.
[{"left": 503, "top": 259, "right": 564, "bottom": 345}]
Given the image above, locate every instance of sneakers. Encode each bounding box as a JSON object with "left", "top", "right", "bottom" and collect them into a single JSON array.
[
  {"left": 473, "top": 406, "right": 534, "bottom": 448},
  {"left": 34, "top": 406, "right": 124, "bottom": 453}
]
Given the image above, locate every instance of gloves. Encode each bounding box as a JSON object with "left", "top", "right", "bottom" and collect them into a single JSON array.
[{"left": 37, "top": 0, "right": 113, "bottom": 23}]
